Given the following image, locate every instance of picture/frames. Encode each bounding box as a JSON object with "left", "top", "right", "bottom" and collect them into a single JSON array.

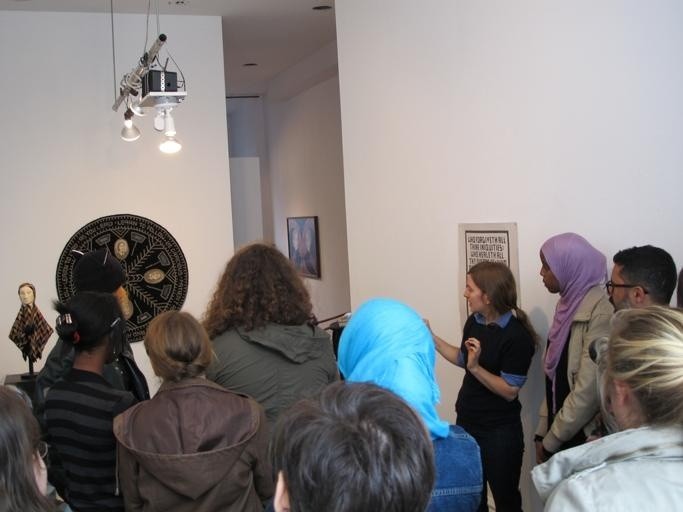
[{"left": 285, "top": 215, "right": 322, "bottom": 280}]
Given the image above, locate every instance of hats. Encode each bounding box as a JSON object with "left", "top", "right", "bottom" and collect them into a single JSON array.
[{"left": 72, "top": 253, "right": 127, "bottom": 296}]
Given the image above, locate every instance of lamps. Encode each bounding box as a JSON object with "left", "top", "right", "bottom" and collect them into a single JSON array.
[{"left": 121, "top": 106, "right": 183, "bottom": 156}]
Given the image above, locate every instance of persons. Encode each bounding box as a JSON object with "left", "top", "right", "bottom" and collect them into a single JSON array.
[
  {"left": 422, "top": 261, "right": 544, "bottom": 512},
  {"left": 112, "top": 311, "right": 273, "bottom": 512},
  {"left": 42, "top": 291, "right": 139, "bottom": 512},
  {"left": 33, "top": 250, "right": 151, "bottom": 402},
  {"left": 0, "top": 383, "right": 73, "bottom": 512},
  {"left": 337, "top": 297, "right": 484, "bottom": 512},
  {"left": 201, "top": 243, "right": 342, "bottom": 430},
  {"left": 530, "top": 304, "right": 683, "bottom": 512},
  {"left": 262, "top": 381, "right": 436, "bottom": 512},
  {"left": 8, "top": 283, "right": 54, "bottom": 364},
  {"left": 584, "top": 245, "right": 677, "bottom": 442},
  {"left": 533, "top": 232, "right": 615, "bottom": 464}
]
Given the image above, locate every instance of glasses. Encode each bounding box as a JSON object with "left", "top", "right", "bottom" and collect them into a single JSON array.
[{"left": 606, "top": 280, "right": 648, "bottom": 296}]
